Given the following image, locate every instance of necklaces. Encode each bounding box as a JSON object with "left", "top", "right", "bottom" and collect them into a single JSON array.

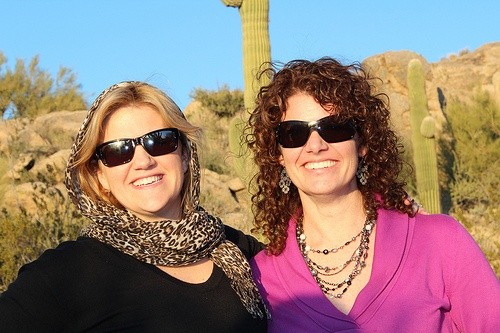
[{"left": 294, "top": 206, "right": 380, "bottom": 300}]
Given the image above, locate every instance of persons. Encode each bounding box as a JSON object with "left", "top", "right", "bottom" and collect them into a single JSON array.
[
  {"left": 242, "top": 52, "right": 500, "bottom": 332},
  {"left": 1, "top": 79, "right": 274, "bottom": 333}
]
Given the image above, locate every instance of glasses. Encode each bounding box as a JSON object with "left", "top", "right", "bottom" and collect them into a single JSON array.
[
  {"left": 93, "top": 127, "right": 187, "bottom": 168},
  {"left": 273, "top": 114, "right": 360, "bottom": 148}
]
